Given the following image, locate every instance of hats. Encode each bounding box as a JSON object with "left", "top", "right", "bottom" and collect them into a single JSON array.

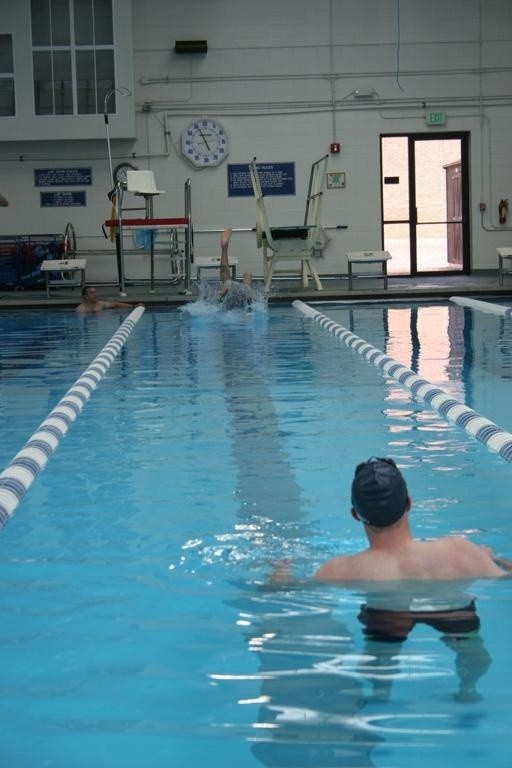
[{"left": 351, "top": 460, "right": 408, "bottom": 527}]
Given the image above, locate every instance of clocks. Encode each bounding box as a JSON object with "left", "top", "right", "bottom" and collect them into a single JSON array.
[
  {"left": 114, "top": 163, "right": 137, "bottom": 188},
  {"left": 180, "top": 119, "right": 230, "bottom": 168}
]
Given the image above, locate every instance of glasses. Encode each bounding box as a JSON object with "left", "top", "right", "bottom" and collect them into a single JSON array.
[{"left": 355, "top": 455, "right": 397, "bottom": 478}]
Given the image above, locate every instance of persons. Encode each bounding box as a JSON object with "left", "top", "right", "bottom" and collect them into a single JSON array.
[
  {"left": 72, "top": 285, "right": 144, "bottom": 314},
  {"left": 264, "top": 454, "right": 512, "bottom": 704},
  {"left": 215, "top": 226, "right": 256, "bottom": 319}
]
